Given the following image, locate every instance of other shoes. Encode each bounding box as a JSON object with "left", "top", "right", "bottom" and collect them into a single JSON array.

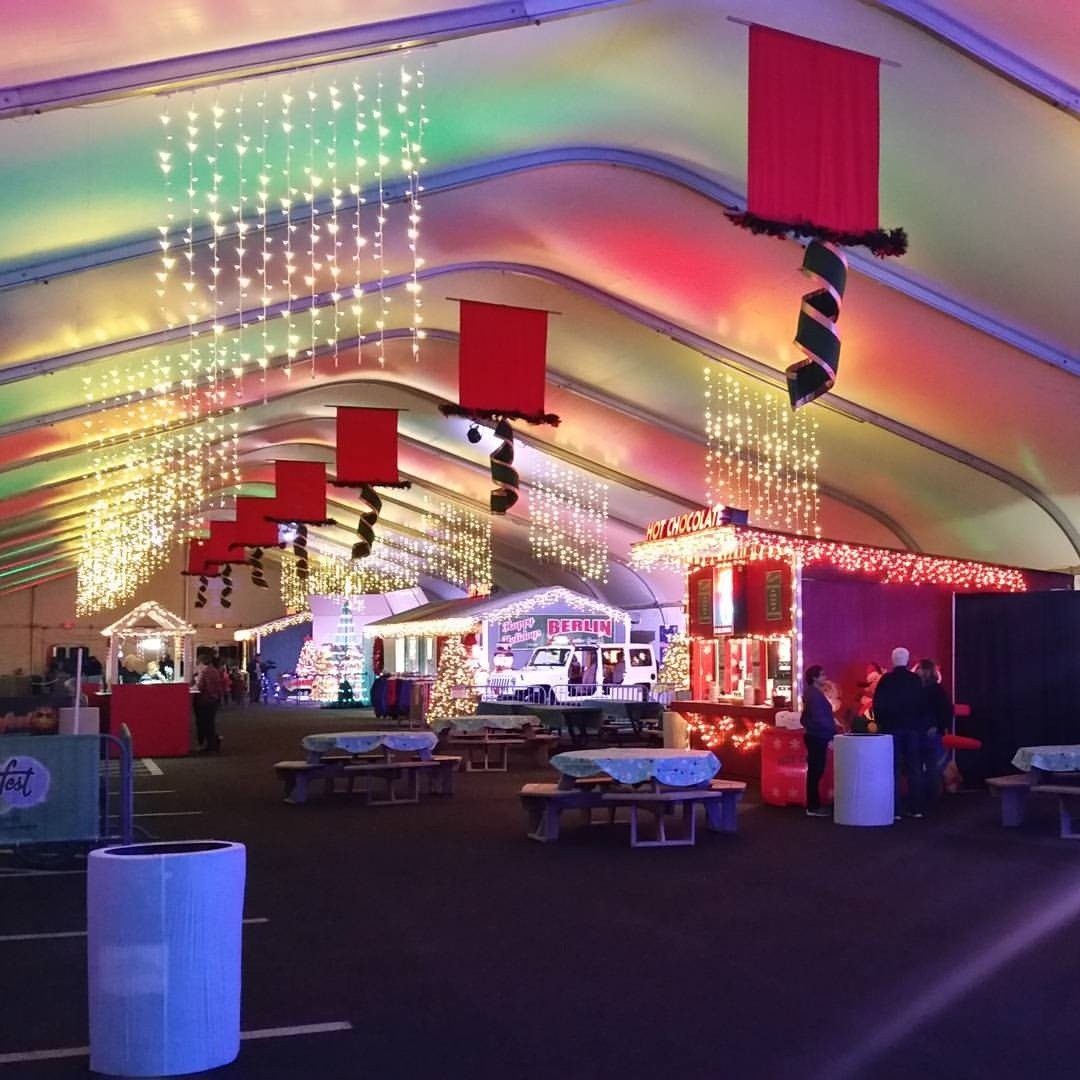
[
  {"left": 192, "top": 743, "right": 220, "bottom": 754},
  {"left": 893, "top": 813, "right": 922, "bottom": 821}
]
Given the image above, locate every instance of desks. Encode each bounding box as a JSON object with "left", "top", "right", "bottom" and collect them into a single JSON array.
[
  {"left": 1011, "top": 740, "right": 1080, "bottom": 837},
  {"left": 434, "top": 712, "right": 546, "bottom": 774}
]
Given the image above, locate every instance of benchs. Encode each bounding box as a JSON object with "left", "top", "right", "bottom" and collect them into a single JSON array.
[
  {"left": 277, "top": 727, "right": 746, "bottom": 850},
  {"left": 1031, "top": 782, "right": 1080, "bottom": 838},
  {"left": 985, "top": 770, "right": 1050, "bottom": 831}
]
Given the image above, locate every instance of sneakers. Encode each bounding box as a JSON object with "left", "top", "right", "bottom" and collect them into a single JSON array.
[{"left": 806, "top": 806, "right": 832, "bottom": 816}]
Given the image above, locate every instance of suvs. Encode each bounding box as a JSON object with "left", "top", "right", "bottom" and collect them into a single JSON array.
[{"left": 490, "top": 644, "right": 658, "bottom": 704}]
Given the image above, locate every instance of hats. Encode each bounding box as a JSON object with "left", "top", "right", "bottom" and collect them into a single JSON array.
[{"left": 892, "top": 647, "right": 910, "bottom": 667}]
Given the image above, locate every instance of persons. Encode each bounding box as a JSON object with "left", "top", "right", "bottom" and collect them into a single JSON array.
[
  {"left": 212, "top": 650, "right": 244, "bottom": 704},
  {"left": 193, "top": 655, "right": 208, "bottom": 743},
  {"left": 45, "top": 654, "right": 102, "bottom": 676},
  {"left": 119, "top": 654, "right": 142, "bottom": 683},
  {"left": 801, "top": 664, "right": 835, "bottom": 816},
  {"left": 141, "top": 660, "right": 165, "bottom": 680},
  {"left": 916, "top": 659, "right": 954, "bottom": 813},
  {"left": 849, "top": 712, "right": 878, "bottom": 732},
  {"left": 198, "top": 655, "right": 220, "bottom": 750},
  {"left": 246, "top": 654, "right": 264, "bottom": 703},
  {"left": 160, "top": 653, "right": 173, "bottom": 675},
  {"left": 874, "top": 647, "right": 932, "bottom": 820}
]
